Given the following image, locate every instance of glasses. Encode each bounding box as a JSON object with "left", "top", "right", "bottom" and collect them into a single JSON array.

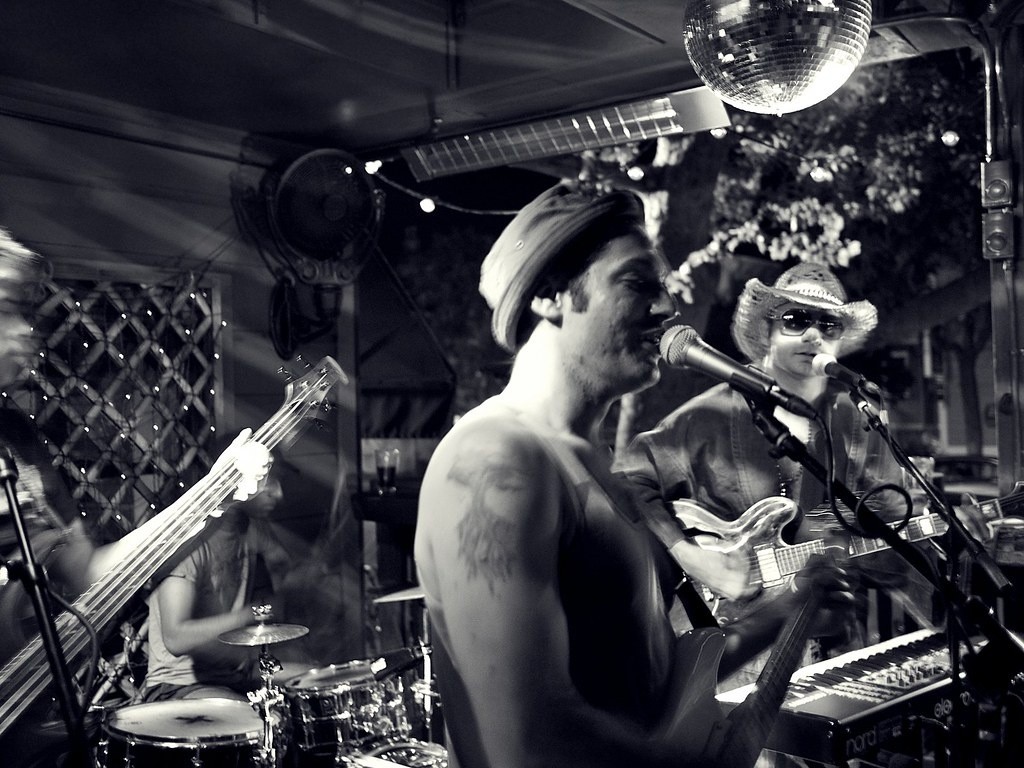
[{"left": 768, "top": 308, "right": 845, "bottom": 342}]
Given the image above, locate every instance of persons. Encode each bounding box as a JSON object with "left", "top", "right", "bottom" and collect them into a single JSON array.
[
  {"left": 622, "top": 263, "right": 992, "bottom": 664},
  {"left": 0, "top": 230, "right": 274, "bottom": 673},
  {"left": 139, "top": 433, "right": 323, "bottom": 701},
  {"left": 413, "top": 184, "right": 855, "bottom": 768}
]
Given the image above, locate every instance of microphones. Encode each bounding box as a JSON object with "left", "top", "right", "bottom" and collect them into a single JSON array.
[
  {"left": 659, "top": 325, "right": 817, "bottom": 420},
  {"left": 811, "top": 352, "right": 882, "bottom": 404}
]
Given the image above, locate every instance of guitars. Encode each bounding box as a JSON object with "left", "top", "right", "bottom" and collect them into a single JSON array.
[
  {"left": 655, "top": 498, "right": 1001, "bottom": 640},
  {"left": 1, "top": 348, "right": 350, "bottom": 768}
]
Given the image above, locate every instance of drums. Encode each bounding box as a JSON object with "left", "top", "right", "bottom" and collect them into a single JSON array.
[
  {"left": 285, "top": 659, "right": 410, "bottom": 756},
  {"left": 99, "top": 698, "right": 289, "bottom": 768},
  {"left": 347, "top": 738, "right": 449, "bottom": 768}
]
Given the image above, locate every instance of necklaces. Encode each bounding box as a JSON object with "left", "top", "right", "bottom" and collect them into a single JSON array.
[{"left": 773, "top": 418, "right": 813, "bottom": 500}]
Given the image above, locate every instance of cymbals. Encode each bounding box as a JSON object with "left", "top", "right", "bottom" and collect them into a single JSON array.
[
  {"left": 218, "top": 620, "right": 309, "bottom": 647},
  {"left": 374, "top": 588, "right": 424, "bottom": 604}
]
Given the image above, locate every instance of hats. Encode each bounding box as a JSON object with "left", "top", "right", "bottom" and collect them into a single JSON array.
[
  {"left": 732, "top": 262, "right": 878, "bottom": 360},
  {"left": 479, "top": 184, "right": 643, "bottom": 350}
]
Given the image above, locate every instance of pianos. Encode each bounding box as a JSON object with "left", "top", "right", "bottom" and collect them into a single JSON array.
[{"left": 717, "top": 623, "right": 1024, "bottom": 768}]
